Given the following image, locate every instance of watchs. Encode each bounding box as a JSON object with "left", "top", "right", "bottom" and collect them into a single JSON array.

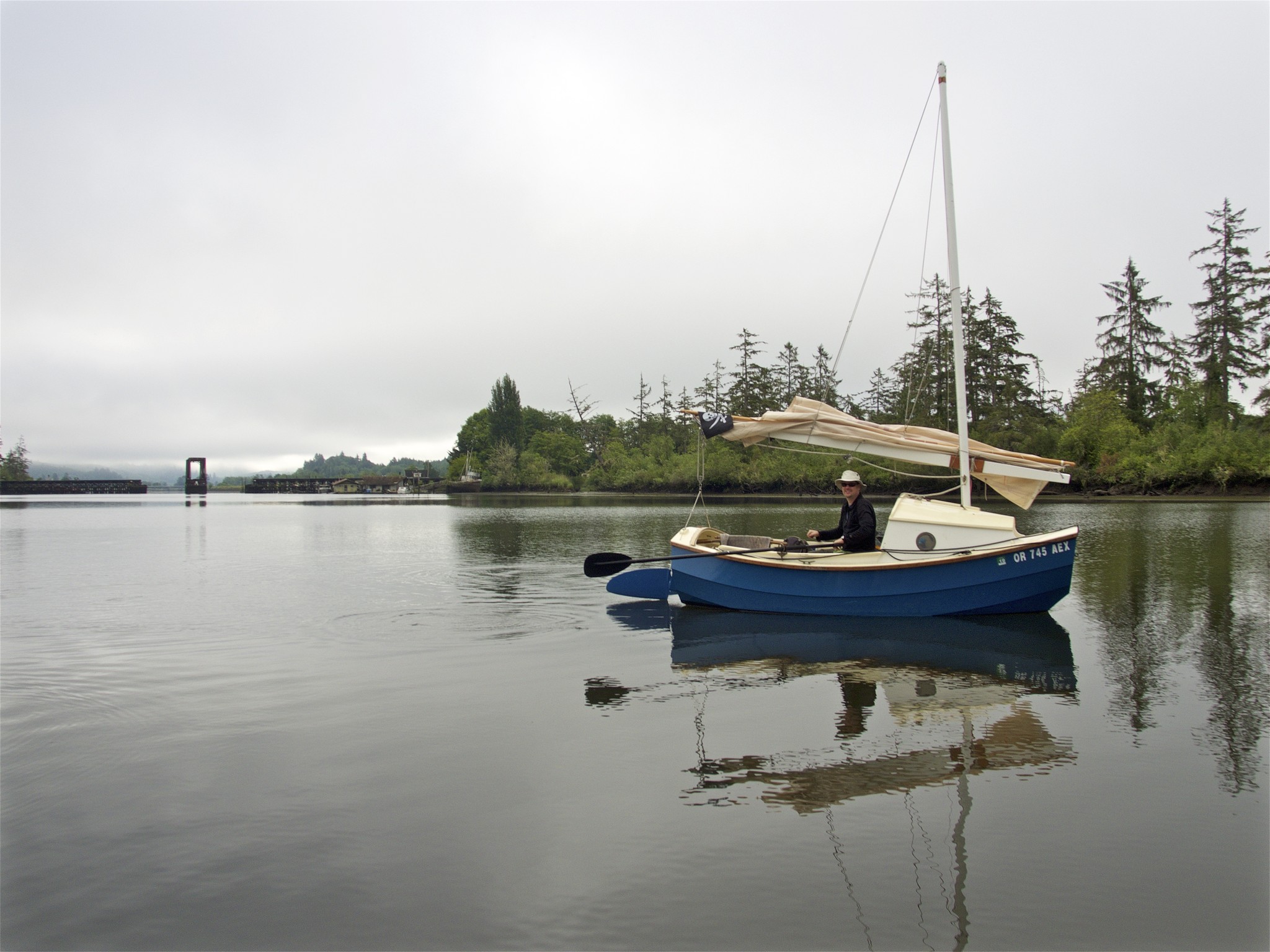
[{"left": 841, "top": 535, "right": 845, "bottom": 541}]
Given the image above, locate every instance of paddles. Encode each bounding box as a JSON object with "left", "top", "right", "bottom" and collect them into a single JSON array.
[{"left": 583, "top": 543, "right": 843, "bottom": 578}]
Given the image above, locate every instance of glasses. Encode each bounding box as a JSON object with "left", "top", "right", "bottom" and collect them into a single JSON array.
[{"left": 840, "top": 482, "right": 860, "bottom": 487}]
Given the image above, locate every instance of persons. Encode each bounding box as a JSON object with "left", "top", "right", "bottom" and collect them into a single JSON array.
[{"left": 807, "top": 470, "right": 876, "bottom": 552}]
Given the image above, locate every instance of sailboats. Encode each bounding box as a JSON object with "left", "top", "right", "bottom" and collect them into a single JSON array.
[{"left": 602, "top": 59, "right": 1082, "bottom": 620}]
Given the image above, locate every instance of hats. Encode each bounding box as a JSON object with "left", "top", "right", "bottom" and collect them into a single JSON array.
[{"left": 835, "top": 470, "right": 867, "bottom": 493}]
